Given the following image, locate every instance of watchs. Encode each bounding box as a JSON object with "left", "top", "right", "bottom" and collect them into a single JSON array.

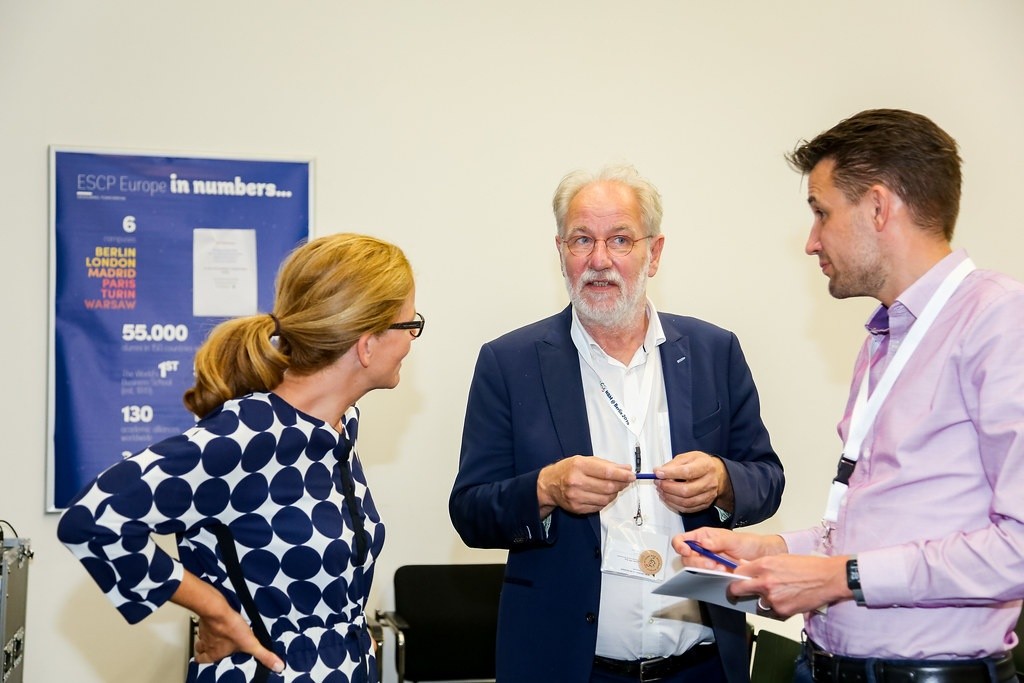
[{"left": 846, "top": 560, "right": 867, "bottom": 607}]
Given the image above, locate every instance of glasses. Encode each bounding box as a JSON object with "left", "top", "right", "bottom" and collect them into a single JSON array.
[
  {"left": 561, "top": 234, "right": 653, "bottom": 257},
  {"left": 387, "top": 313, "right": 425, "bottom": 337}
]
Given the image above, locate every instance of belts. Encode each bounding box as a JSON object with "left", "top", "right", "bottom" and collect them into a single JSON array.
[
  {"left": 801, "top": 636, "right": 1018, "bottom": 683},
  {"left": 591, "top": 643, "right": 713, "bottom": 681}
]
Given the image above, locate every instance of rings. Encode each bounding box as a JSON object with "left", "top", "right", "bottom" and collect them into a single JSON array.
[{"left": 758, "top": 598, "right": 770, "bottom": 611}]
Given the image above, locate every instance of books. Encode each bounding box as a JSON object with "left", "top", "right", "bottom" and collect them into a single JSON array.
[{"left": 652, "top": 567, "right": 791, "bottom": 622}]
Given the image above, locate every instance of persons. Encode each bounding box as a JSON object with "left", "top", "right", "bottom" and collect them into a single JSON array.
[
  {"left": 448, "top": 165, "right": 786, "bottom": 683},
  {"left": 57, "top": 233, "right": 425, "bottom": 683},
  {"left": 670, "top": 109, "right": 1024, "bottom": 683}
]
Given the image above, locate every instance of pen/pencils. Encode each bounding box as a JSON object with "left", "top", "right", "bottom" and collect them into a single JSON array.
[
  {"left": 636, "top": 473, "right": 660, "bottom": 479},
  {"left": 684, "top": 540, "right": 739, "bottom": 568}
]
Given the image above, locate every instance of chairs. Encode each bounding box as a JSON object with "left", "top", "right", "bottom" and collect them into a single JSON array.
[{"left": 376, "top": 565, "right": 505, "bottom": 683}]
